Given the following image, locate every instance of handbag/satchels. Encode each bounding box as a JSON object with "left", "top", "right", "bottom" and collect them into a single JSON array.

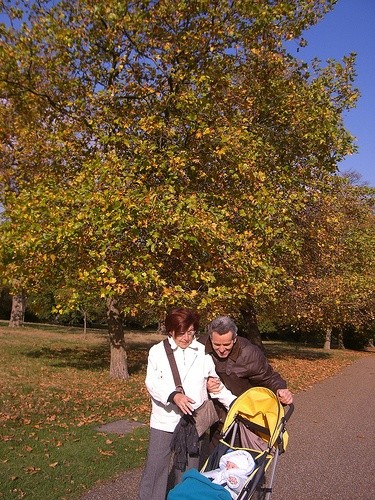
[{"left": 192, "top": 398, "right": 219, "bottom": 437}]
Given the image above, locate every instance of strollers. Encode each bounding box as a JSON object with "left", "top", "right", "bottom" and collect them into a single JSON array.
[{"left": 167, "top": 387, "right": 295, "bottom": 500}]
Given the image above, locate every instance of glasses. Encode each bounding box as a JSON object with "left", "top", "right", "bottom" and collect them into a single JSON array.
[{"left": 177, "top": 330, "right": 196, "bottom": 336}]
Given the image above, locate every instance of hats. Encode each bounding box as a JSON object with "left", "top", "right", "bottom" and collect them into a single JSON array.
[{"left": 227, "top": 455, "right": 247, "bottom": 468}]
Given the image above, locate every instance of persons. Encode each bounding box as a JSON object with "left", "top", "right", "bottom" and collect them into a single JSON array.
[
  {"left": 136, "top": 308, "right": 238, "bottom": 500},
  {"left": 196, "top": 317, "right": 293, "bottom": 473},
  {"left": 203, "top": 450, "right": 255, "bottom": 500}
]
